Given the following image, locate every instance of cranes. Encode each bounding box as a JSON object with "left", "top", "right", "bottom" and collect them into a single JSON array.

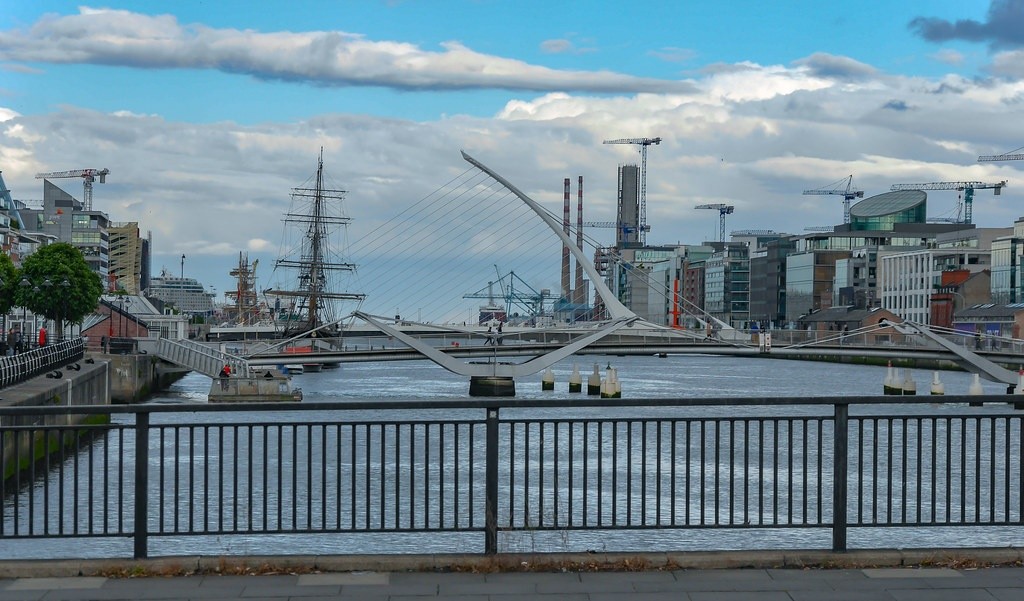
[
  {"left": 34, "top": 168, "right": 110, "bottom": 212},
  {"left": 603, "top": 136, "right": 663, "bottom": 247},
  {"left": 891, "top": 180, "right": 1006, "bottom": 226},
  {"left": 694, "top": 203, "right": 734, "bottom": 242},
  {"left": 462, "top": 271, "right": 561, "bottom": 314},
  {"left": 565, "top": 221, "right": 640, "bottom": 242},
  {"left": 803, "top": 175, "right": 864, "bottom": 224},
  {"left": 977, "top": 147, "right": 1024, "bottom": 162}
]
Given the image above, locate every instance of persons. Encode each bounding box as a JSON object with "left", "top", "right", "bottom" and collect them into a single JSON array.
[
  {"left": 991, "top": 333, "right": 1000, "bottom": 351},
  {"left": 841, "top": 324, "right": 850, "bottom": 345},
  {"left": 493, "top": 322, "right": 503, "bottom": 344},
  {"left": 484, "top": 327, "right": 493, "bottom": 345},
  {"left": 100, "top": 335, "right": 107, "bottom": 354},
  {"left": 82, "top": 334, "right": 88, "bottom": 353},
  {"left": 703, "top": 320, "right": 714, "bottom": 342},
  {"left": 264, "top": 371, "right": 274, "bottom": 377},
  {"left": 975, "top": 329, "right": 983, "bottom": 351},
  {"left": 219, "top": 364, "right": 231, "bottom": 392},
  {"left": 6, "top": 328, "right": 16, "bottom": 356}
]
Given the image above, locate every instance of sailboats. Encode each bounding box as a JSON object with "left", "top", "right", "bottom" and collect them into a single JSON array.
[{"left": 262, "top": 146, "right": 369, "bottom": 372}]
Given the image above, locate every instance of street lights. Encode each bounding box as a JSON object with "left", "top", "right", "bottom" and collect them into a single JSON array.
[
  {"left": 60, "top": 278, "right": 71, "bottom": 341},
  {"left": 41, "top": 279, "right": 53, "bottom": 346},
  {"left": 122, "top": 296, "right": 133, "bottom": 337},
  {"left": 115, "top": 293, "right": 124, "bottom": 337},
  {"left": 18, "top": 276, "right": 31, "bottom": 351},
  {"left": 106, "top": 290, "right": 117, "bottom": 336}
]
